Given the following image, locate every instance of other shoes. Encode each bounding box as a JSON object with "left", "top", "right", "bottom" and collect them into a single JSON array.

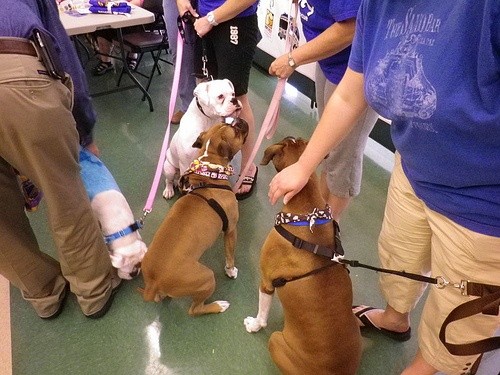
[
  {"left": 168, "top": 110, "right": 185, "bottom": 125},
  {"left": 85, "top": 278, "right": 124, "bottom": 319}
]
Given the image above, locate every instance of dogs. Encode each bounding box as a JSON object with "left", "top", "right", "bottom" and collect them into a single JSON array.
[
  {"left": 142, "top": 117, "right": 249, "bottom": 315},
  {"left": 79, "top": 147, "right": 148, "bottom": 280},
  {"left": 162, "top": 78, "right": 243, "bottom": 199},
  {"left": 244, "top": 137, "right": 363, "bottom": 375}
]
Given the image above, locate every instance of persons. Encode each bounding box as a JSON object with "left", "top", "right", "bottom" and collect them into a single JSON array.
[
  {"left": 269, "top": 0, "right": 379, "bottom": 221},
  {"left": 163, "top": 0, "right": 195, "bottom": 123},
  {"left": 268, "top": 0, "right": 500, "bottom": 375},
  {"left": 0, "top": 1, "right": 124, "bottom": 319},
  {"left": 177, "top": 0, "right": 259, "bottom": 200},
  {"left": 91, "top": 0, "right": 161, "bottom": 76}
]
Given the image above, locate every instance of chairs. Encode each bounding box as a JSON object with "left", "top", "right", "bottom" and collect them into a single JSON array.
[{"left": 116, "top": 0, "right": 169, "bottom": 102}]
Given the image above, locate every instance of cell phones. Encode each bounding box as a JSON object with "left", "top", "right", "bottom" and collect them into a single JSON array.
[{"left": 36, "top": 31, "right": 61, "bottom": 78}]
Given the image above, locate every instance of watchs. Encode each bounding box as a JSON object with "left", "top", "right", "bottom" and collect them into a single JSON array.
[
  {"left": 207, "top": 11, "right": 218, "bottom": 26},
  {"left": 288, "top": 52, "right": 298, "bottom": 69}
]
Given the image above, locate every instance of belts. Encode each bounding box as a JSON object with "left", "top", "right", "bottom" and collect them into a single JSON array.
[{"left": 0, "top": 40, "right": 37, "bottom": 56}]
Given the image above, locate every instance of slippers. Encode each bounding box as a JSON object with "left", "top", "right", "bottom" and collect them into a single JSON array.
[{"left": 234, "top": 165, "right": 258, "bottom": 200}]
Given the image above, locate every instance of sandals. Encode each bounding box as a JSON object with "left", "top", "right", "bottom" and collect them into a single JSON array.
[
  {"left": 352, "top": 305, "right": 411, "bottom": 342},
  {"left": 125, "top": 51, "right": 138, "bottom": 71},
  {"left": 94, "top": 61, "right": 113, "bottom": 75}
]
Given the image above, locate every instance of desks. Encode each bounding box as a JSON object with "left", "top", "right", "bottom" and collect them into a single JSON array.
[{"left": 57, "top": 0, "right": 155, "bottom": 113}]
